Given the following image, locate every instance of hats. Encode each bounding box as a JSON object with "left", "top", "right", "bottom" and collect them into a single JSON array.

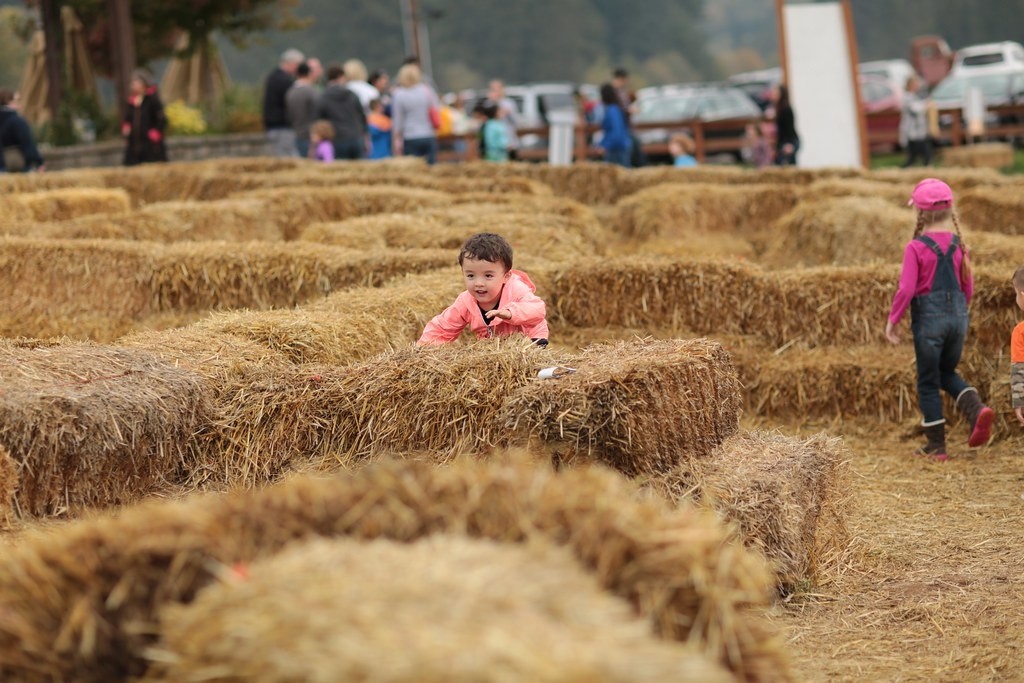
[{"left": 907, "top": 178, "right": 953, "bottom": 210}]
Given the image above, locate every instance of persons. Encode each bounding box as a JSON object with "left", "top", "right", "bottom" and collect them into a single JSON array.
[
  {"left": 262, "top": 48, "right": 638, "bottom": 168},
  {"left": 898, "top": 76, "right": 931, "bottom": 167},
  {"left": 886, "top": 178, "right": 995, "bottom": 459},
  {"left": 0, "top": 86, "right": 43, "bottom": 173},
  {"left": 122, "top": 74, "right": 168, "bottom": 167},
  {"left": 1010, "top": 265, "right": 1024, "bottom": 423},
  {"left": 667, "top": 132, "right": 698, "bottom": 167},
  {"left": 416, "top": 232, "right": 549, "bottom": 348},
  {"left": 772, "top": 85, "right": 800, "bottom": 166},
  {"left": 741, "top": 126, "right": 771, "bottom": 170}
]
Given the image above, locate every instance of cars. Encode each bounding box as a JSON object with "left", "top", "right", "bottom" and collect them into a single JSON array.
[{"left": 427, "top": 35, "right": 1022, "bottom": 167}]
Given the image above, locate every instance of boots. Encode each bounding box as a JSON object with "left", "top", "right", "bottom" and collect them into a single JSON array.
[
  {"left": 954, "top": 385, "right": 995, "bottom": 447},
  {"left": 913, "top": 419, "right": 949, "bottom": 460}
]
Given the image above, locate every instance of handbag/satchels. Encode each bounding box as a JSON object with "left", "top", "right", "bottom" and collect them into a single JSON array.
[{"left": 435, "top": 106, "right": 454, "bottom": 139}]
